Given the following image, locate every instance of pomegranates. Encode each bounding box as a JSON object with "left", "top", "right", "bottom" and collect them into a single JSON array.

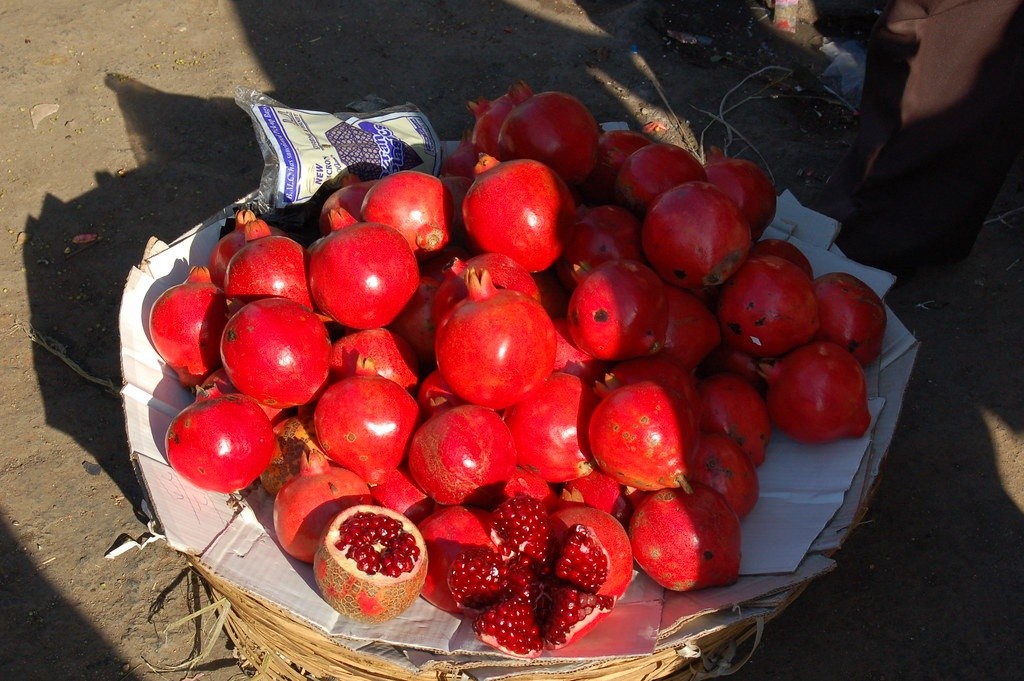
[{"left": 148, "top": 84, "right": 888, "bottom": 659}]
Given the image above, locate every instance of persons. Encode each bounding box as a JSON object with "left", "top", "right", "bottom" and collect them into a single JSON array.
[{"left": 821, "top": 1, "right": 1022, "bottom": 280}]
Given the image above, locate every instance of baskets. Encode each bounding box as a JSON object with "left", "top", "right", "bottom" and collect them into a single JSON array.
[{"left": 117, "top": 141, "right": 922, "bottom": 681}]
